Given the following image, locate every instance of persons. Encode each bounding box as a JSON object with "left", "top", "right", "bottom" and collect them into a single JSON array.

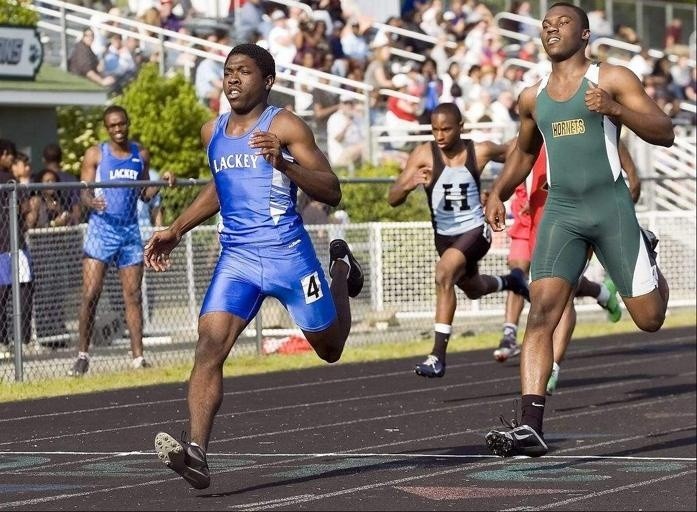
[
  {"left": 389, "top": 104, "right": 530, "bottom": 378},
  {"left": 135, "top": 44, "right": 369, "bottom": 489},
  {"left": 0, "top": 138, "right": 86, "bottom": 355},
  {"left": 494, "top": 138, "right": 642, "bottom": 398},
  {"left": 71, "top": 0, "right": 552, "bottom": 169},
  {"left": 300, "top": 194, "right": 330, "bottom": 264},
  {"left": 481, "top": 2, "right": 675, "bottom": 456},
  {"left": 586, "top": 4, "right": 697, "bottom": 125},
  {"left": 68, "top": 106, "right": 175, "bottom": 378}
]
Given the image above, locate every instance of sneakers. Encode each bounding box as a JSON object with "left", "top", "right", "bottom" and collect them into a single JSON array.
[
  {"left": 506, "top": 267, "right": 533, "bottom": 303},
  {"left": 600, "top": 276, "right": 622, "bottom": 324},
  {"left": 485, "top": 416, "right": 548, "bottom": 456},
  {"left": 415, "top": 355, "right": 447, "bottom": 377},
  {"left": 327, "top": 236, "right": 365, "bottom": 298},
  {"left": 67, "top": 353, "right": 89, "bottom": 377},
  {"left": 131, "top": 355, "right": 151, "bottom": 369},
  {"left": 546, "top": 362, "right": 559, "bottom": 395},
  {"left": 640, "top": 226, "right": 662, "bottom": 253},
  {"left": 493, "top": 328, "right": 521, "bottom": 362},
  {"left": 153, "top": 427, "right": 212, "bottom": 493}
]
